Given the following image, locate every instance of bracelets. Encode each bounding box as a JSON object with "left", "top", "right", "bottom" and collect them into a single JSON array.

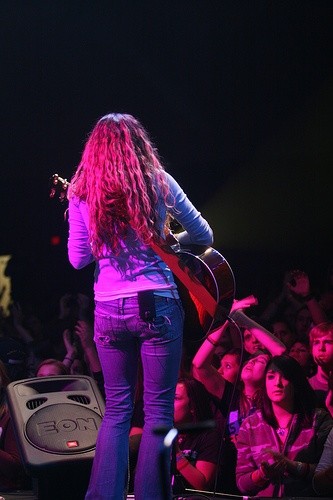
[
  {"left": 64, "top": 356, "right": 73, "bottom": 363},
  {"left": 302, "top": 293, "right": 314, "bottom": 302},
  {"left": 205, "top": 335, "right": 219, "bottom": 347},
  {"left": 247, "top": 320, "right": 255, "bottom": 330}
]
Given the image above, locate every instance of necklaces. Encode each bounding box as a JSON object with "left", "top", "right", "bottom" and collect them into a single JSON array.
[{"left": 276, "top": 414, "right": 294, "bottom": 435}]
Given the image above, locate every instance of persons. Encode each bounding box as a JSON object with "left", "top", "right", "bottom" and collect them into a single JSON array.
[
  {"left": 67, "top": 112, "right": 214, "bottom": 500},
  {"left": 0, "top": 267, "right": 333, "bottom": 500}
]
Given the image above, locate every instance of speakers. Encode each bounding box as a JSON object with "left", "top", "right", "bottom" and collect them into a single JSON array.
[{"left": 6, "top": 375, "right": 106, "bottom": 499}]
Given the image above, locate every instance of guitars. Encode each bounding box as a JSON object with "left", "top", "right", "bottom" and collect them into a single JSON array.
[{"left": 48, "top": 174, "right": 236, "bottom": 342}]
